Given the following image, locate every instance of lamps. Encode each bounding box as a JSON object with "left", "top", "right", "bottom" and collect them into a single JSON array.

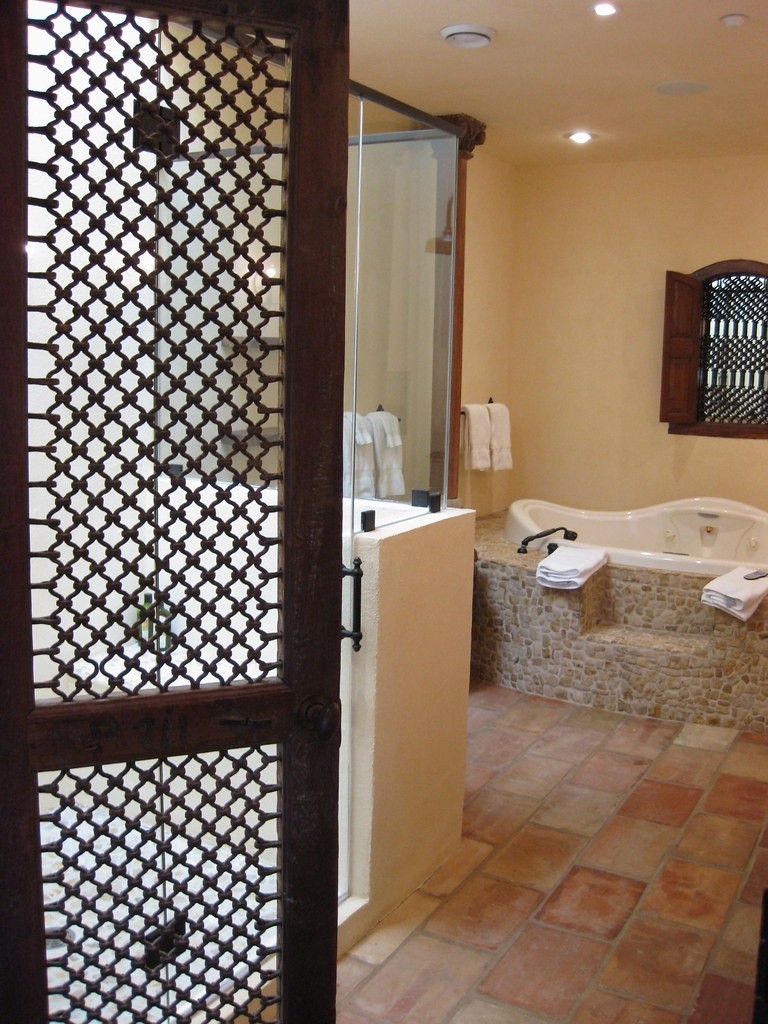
[
  {"left": 655, "top": 80, "right": 705, "bottom": 97},
  {"left": 561, "top": 129, "right": 600, "bottom": 145},
  {"left": 442, "top": 25, "right": 494, "bottom": 48},
  {"left": 720, "top": 14, "right": 747, "bottom": 27}
]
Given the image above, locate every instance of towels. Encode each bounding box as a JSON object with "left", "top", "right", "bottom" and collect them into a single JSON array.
[
  {"left": 484, "top": 403, "right": 512, "bottom": 470},
  {"left": 460, "top": 404, "right": 491, "bottom": 472},
  {"left": 366, "top": 412, "right": 405, "bottom": 498},
  {"left": 700, "top": 566, "right": 768, "bottom": 622},
  {"left": 343, "top": 411, "right": 376, "bottom": 500},
  {"left": 535, "top": 546, "right": 608, "bottom": 589}
]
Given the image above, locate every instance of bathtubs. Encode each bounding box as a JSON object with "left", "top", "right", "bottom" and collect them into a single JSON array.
[{"left": 503, "top": 496, "right": 768, "bottom": 576}]
